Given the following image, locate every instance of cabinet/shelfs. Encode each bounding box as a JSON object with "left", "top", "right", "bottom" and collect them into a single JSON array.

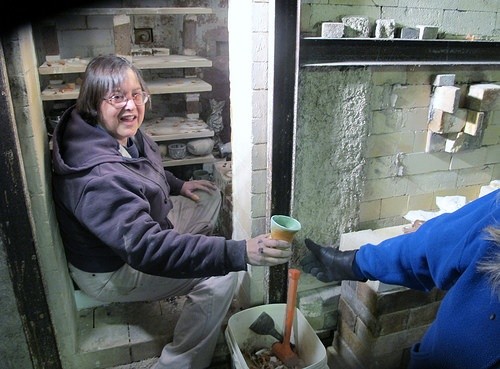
[{"left": 39, "top": 8, "right": 214, "bottom": 167}]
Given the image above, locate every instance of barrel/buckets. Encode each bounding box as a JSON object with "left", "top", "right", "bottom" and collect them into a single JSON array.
[{"left": 225, "top": 303, "right": 328, "bottom": 369}]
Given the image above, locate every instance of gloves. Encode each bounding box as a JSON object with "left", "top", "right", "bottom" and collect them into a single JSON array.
[{"left": 298, "top": 238, "right": 368, "bottom": 283}]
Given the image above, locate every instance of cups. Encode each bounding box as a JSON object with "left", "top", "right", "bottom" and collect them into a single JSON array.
[
  {"left": 270, "top": 215, "right": 301, "bottom": 251},
  {"left": 168, "top": 144, "right": 187, "bottom": 159},
  {"left": 192, "top": 170, "right": 211, "bottom": 181}
]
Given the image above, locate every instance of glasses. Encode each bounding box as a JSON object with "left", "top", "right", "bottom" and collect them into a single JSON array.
[{"left": 98, "top": 92, "right": 150, "bottom": 109}]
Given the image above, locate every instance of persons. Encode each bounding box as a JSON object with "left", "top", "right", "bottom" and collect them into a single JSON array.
[
  {"left": 300, "top": 189, "right": 499, "bottom": 369},
  {"left": 53, "top": 53, "right": 293, "bottom": 369}
]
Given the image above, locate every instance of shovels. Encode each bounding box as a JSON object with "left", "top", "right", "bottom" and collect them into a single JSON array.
[{"left": 250, "top": 310, "right": 295, "bottom": 348}]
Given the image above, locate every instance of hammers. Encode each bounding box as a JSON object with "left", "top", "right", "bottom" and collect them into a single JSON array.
[{"left": 272, "top": 268, "right": 300, "bottom": 367}]
